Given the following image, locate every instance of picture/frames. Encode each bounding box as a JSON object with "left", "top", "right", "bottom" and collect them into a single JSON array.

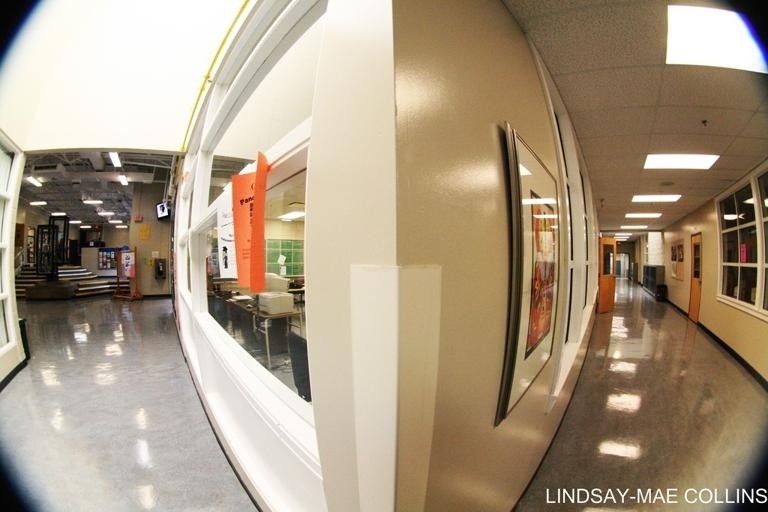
[{"left": 494, "top": 120, "right": 560, "bottom": 427}]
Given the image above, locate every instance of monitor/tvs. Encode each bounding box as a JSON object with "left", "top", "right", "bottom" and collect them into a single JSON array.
[{"left": 155, "top": 201, "right": 171, "bottom": 221}]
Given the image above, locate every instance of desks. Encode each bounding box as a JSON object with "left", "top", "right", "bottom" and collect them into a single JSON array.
[{"left": 228, "top": 297, "right": 303, "bottom": 370}]
